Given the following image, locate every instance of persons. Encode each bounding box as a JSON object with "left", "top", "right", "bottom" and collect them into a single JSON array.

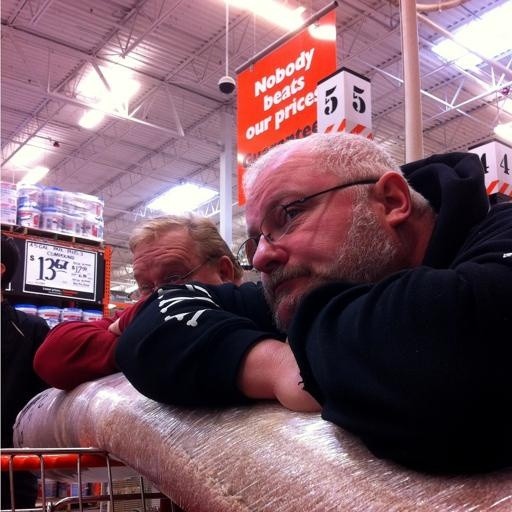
[
  {"left": 31, "top": 208, "right": 246, "bottom": 393},
  {"left": 112, "top": 131, "right": 512, "bottom": 474},
  {"left": 1, "top": 231, "right": 50, "bottom": 509}
]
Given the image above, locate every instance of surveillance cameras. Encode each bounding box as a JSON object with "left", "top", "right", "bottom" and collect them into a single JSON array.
[{"left": 218, "top": 76, "right": 235, "bottom": 93}]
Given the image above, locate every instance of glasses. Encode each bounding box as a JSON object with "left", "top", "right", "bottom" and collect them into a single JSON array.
[
  {"left": 127, "top": 254, "right": 217, "bottom": 304},
  {"left": 236, "top": 179, "right": 380, "bottom": 271}
]
